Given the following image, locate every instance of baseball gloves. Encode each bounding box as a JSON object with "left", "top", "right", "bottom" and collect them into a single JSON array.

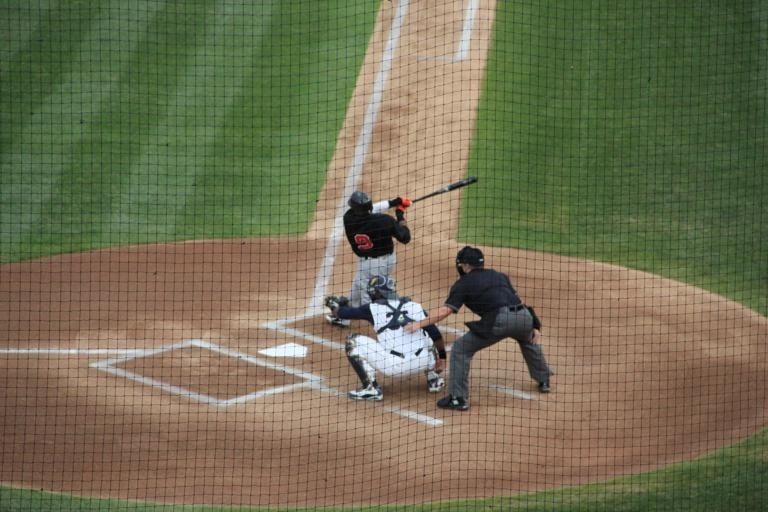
[{"left": 325, "top": 295, "right": 349, "bottom": 314}]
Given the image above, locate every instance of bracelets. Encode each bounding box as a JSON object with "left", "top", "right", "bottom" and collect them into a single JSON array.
[{"left": 439, "top": 352, "right": 446, "bottom": 359}]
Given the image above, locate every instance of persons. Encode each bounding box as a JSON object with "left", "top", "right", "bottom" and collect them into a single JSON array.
[
  {"left": 324, "top": 192, "right": 412, "bottom": 327},
  {"left": 325, "top": 275, "right": 446, "bottom": 401},
  {"left": 404, "top": 245, "right": 554, "bottom": 410}
]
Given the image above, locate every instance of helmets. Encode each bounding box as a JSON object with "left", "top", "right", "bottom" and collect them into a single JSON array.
[
  {"left": 367, "top": 276, "right": 395, "bottom": 299},
  {"left": 348, "top": 192, "right": 371, "bottom": 208},
  {"left": 457, "top": 246, "right": 484, "bottom": 267}
]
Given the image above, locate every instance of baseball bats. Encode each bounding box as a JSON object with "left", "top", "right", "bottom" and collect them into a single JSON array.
[{"left": 403, "top": 175, "right": 478, "bottom": 209}]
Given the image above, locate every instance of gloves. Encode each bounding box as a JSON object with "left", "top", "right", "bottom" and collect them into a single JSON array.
[{"left": 388, "top": 197, "right": 410, "bottom": 221}]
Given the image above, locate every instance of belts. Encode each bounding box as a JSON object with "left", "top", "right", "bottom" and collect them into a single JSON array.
[
  {"left": 391, "top": 348, "right": 422, "bottom": 357},
  {"left": 510, "top": 305, "right": 523, "bottom": 311}
]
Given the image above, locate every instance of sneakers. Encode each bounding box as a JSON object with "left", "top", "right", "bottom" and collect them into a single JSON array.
[
  {"left": 350, "top": 384, "right": 383, "bottom": 401},
  {"left": 539, "top": 379, "right": 549, "bottom": 392},
  {"left": 326, "top": 315, "right": 350, "bottom": 327},
  {"left": 427, "top": 376, "right": 445, "bottom": 392},
  {"left": 438, "top": 394, "right": 468, "bottom": 409}
]
